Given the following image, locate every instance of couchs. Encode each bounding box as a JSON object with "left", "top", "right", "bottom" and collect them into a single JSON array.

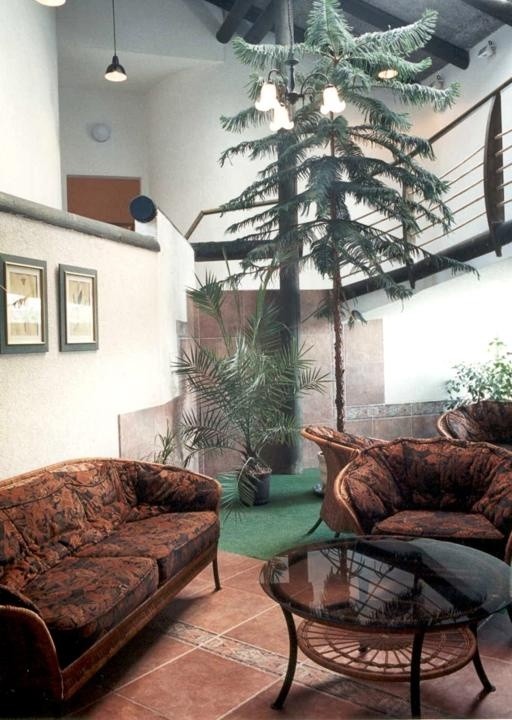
[
  {"left": 1, "top": 455, "right": 223, "bottom": 707},
  {"left": 300, "top": 424, "right": 392, "bottom": 540},
  {"left": 434, "top": 398, "right": 512, "bottom": 459},
  {"left": 334, "top": 435, "right": 512, "bottom": 561}
]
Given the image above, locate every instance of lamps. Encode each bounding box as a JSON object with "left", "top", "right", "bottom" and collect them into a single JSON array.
[
  {"left": 248, "top": 1, "right": 347, "bottom": 132},
  {"left": 104, "top": 1, "right": 130, "bottom": 83}
]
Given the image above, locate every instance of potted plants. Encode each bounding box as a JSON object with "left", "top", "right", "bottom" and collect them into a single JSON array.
[{"left": 151, "top": 246, "right": 336, "bottom": 508}]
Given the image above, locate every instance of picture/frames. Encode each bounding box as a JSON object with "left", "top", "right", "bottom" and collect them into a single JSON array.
[
  {"left": 0, "top": 251, "right": 50, "bottom": 355},
  {"left": 59, "top": 262, "right": 100, "bottom": 353}
]
